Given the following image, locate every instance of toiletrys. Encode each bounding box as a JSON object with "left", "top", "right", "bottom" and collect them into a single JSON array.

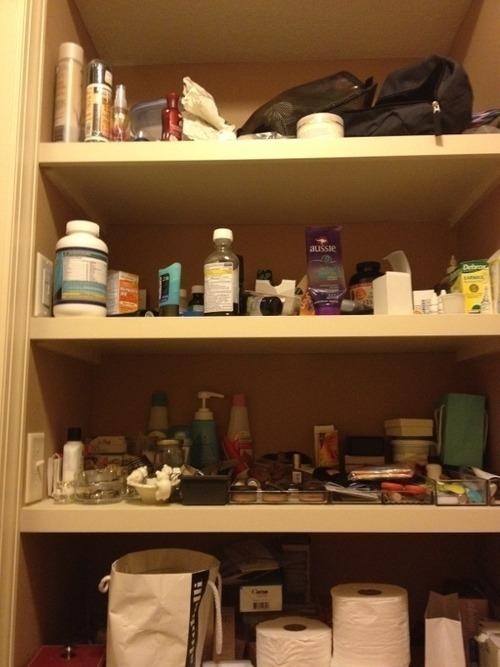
[
  {"left": 304, "top": 222, "right": 348, "bottom": 316},
  {"left": 295, "top": 111, "right": 346, "bottom": 138},
  {"left": 157, "top": 262, "right": 182, "bottom": 316},
  {"left": 110, "top": 81, "right": 130, "bottom": 142}
]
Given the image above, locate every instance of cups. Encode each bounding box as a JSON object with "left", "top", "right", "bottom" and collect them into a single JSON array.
[{"left": 62, "top": 467, "right": 131, "bottom": 505}]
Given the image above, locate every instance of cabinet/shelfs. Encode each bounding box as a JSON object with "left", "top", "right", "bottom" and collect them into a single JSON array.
[{"left": 1, "top": 0, "right": 500, "bottom": 667}]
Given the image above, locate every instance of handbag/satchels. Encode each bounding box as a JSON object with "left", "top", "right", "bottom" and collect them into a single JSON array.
[
  {"left": 379, "top": 54, "right": 474, "bottom": 135},
  {"left": 237, "top": 70, "right": 378, "bottom": 137},
  {"left": 99, "top": 548, "right": 223, "bottom": 666},
  {"left": 434, "top": 393, "right": 488, "bottom": 468}
]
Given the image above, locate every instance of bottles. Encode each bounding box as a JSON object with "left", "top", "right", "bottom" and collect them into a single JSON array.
[
  {"left": 51, "top": 220, "right": 110, "bottom": 318},
  {"left": 152, "top": 439, "right": 183, "bottom": 475},
  {"left": 147, "top": 391, "right": 171, "bottom": 466},
  {"left": 178, "top": 288, "right": 189, "bottom": 316},
  {"left": 187, "top": 284, "right": 205, "bottom": 316},
  {"left": 202, "top": 227, "right": 242, "bottom": 316},
  {"left": 225, "top": 391, "right": 255, "bottom": 469},
  {"left": 346, "top": 260, "right": 385, "bottom": 315}
]
